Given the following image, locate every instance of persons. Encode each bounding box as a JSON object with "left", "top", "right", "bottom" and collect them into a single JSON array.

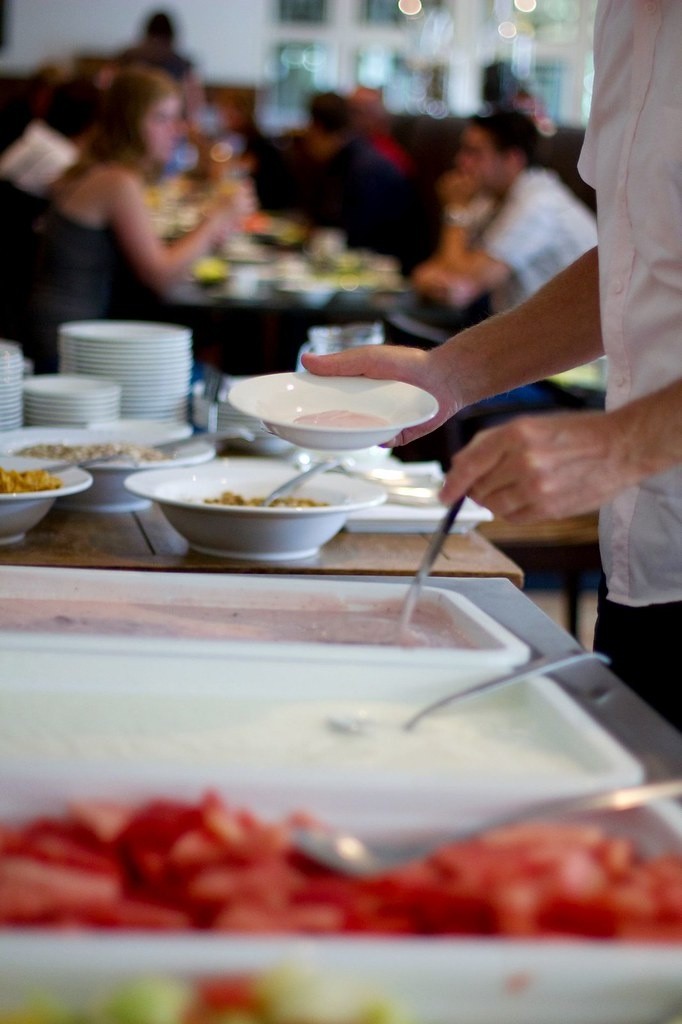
[
  {"left": 301, "top": 0, "right": 682, "bottom": 733},
  {"left": 21, "top": 12, "right": 252, "bottom": 375},
  {"left": 212, "top": 65, "right": 571, "bottom": 283},
  {"left": 404, "top": 111, "right": 608, "bottom": 474}
]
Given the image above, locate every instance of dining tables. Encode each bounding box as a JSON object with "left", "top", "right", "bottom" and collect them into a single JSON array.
[
  {"left": 0, "top": 445, "right": 525, "bottom": 591},
  {"left": 161, "top": 250, "right": 406, "bottom": 375}
]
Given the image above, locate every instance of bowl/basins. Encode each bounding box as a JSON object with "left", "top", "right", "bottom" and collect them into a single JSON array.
[
  {"left": 123, "top": 461, "right": 387, "bottom": 559},
  {"left": 0, "top": 456, "right": 93, "bottom": 544},
  {"left": 0, "top": 418, "right": 215, "bottom": 513},
  {"left": 228, "top": 372, "right": 440, "bottom": 450}
]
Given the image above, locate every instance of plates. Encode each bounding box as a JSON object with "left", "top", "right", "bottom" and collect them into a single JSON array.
[
  {"left": 345, "top": 495, "right": 494, "bottom": 533},
  {"left": 0, "top": 320, "right": 192, "bottom": 431},
  {"left": 193, "top": 381, "right": 290, "bottom": 456}
]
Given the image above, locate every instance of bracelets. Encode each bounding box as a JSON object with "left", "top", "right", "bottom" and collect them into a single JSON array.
[{"left": 444, "top": 213, "right": 468, "bottom": 227}]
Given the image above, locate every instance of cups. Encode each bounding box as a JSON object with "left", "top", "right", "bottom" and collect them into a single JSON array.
[{"left": 297, "top": 322, "right": 384, "bottom": 374}]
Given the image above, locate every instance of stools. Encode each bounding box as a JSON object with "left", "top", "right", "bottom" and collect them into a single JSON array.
[{"left": 475, "top": 516, "right": 596, "bottom": 640}]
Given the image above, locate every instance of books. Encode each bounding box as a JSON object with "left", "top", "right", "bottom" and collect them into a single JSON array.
[{"left": 0, "top": 119, "right": 81, "bottom": 199}]
